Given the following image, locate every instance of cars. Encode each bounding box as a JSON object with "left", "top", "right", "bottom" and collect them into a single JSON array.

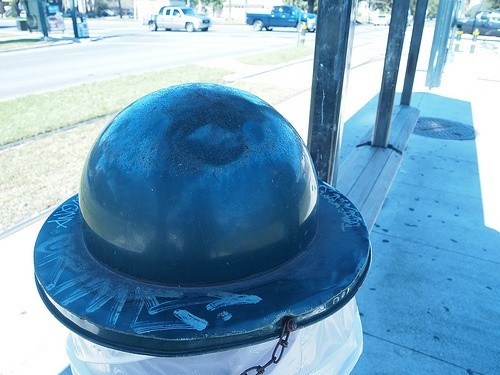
[
  {"left": 373, "top": 13, "right": 391, "bottom": 25},
  {"left": 456, "top": 10, "right": 500, "bottom": 37}
]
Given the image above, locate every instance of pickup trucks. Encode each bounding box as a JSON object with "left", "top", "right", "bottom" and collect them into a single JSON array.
[
  {"left": 245, "top": 5, "right": 318, "bottom": 33},
  {"left": 146, "top": 4, "right": 212, "bottom": 31}
]
